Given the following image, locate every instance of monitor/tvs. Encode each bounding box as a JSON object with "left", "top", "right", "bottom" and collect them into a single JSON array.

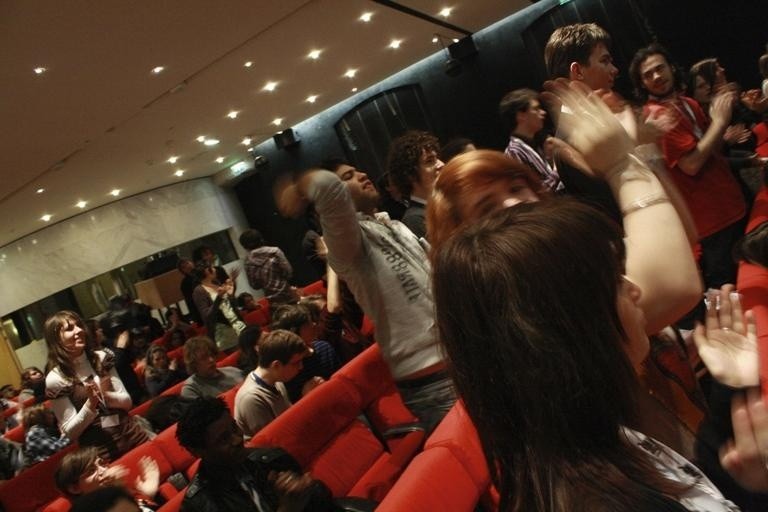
[{"left": 145, "top": 251, "right": 179, "bottom": 277}]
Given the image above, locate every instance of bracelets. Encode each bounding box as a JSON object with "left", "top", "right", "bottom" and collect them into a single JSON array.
[{"left": 621, "top": 194, "right": 668, "bottom": 218}]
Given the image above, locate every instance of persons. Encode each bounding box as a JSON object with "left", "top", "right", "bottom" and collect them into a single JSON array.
[
  {"left": 3, "top": 231, "right": 292, "bottom": 512},
  {"left": 278, "top": 22, "right": 767, "bottom": 512}
]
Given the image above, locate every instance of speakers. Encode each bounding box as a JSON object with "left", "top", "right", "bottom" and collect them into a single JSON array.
[
  {"left": 455, "top": 34, "right": 479, "bottom": 61},
  {"left": 272, "top": 127, "right": 301, "bottom": 150}
]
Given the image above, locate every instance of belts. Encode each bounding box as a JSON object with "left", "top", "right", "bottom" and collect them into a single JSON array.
[{"left": 394, "top": 367, "right": 452, "bottom": 390}]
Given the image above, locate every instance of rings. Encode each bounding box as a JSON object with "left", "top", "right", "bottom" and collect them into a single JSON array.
[{"left": 720, "top": 328, "right": 730, "bottom": 330}]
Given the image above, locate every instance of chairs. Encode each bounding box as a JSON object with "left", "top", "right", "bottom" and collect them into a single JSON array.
[{"left": 0, "top": 123, "right": 768, "bottom": 512}]
